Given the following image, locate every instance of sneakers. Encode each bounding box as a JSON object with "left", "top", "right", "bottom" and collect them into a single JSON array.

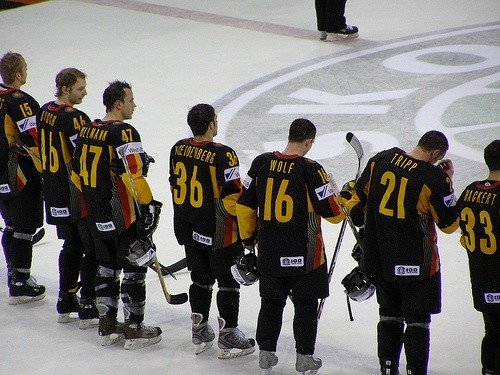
[
  {"left": 78, "top": 298, "right": 100, "bottom": 330},
  {"left": 258, "top": 351, "right": 279, "bottom": 374},
  {"left": 318, "top": 24, "right": 360, "bottom": 42},
  {"left": 190, "top": 313, "right": 217, "bottom": 355},
  {"left": 215, "top": 316, "right": 256, "bottom": 360},
  {"left": 96, "top": 313, "right": 121, "bottom": 349},
  {"left": 56, "top": 291, "right": 79, "bottom": 324},
  {"left": 295, "top": 352, "right": 323, "bottom": 375},
  {"left": 122, "top": 319, "right": 164, "bottom": 351},
  {"left": 6, "top": 268, "right": 47, "bottom": 307}
]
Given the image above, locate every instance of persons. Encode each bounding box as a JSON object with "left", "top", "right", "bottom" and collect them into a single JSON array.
[
  {"left": 346, "top": 130, "right": 460, "bottom": 375},
  {"left": 73, "top": 80, "right": 162, "bottom": 349},
  {"left": 314, "top": 0, "right": 359, "bottom": 41},
  {"left": 169, "top": 104, "right": 257, "bottom": 360},
  {"left": 237, "top": 118, "right": 356, "bottom": 375},
  {"left": 0, "top": 52, "right": 47, "bottom": 305},
  {"left": 457, "top": 140, "right": 500, "bottom": 375},
  {"left": 37, "top": 68, "right": 100, "bottom": 329}
]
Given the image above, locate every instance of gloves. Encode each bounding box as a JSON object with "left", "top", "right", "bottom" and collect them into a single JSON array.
[
  {"left": 339, "top": 180, "right": 357, "bottom": 200},
  {"left": 140, "top": 200, "right": 164, "bottom": 238}
]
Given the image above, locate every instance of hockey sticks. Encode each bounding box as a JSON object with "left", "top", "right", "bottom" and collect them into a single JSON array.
[
  {"left": 148, "top": 253, "right": 188, "bottom": 276},
  {"left": 325, "top": 172, "right": 363, "bottom": 253},
  {"left": 12, "top": 138, "right": 41, "bottom": 162},
  {"left": 318, "top": 132, "right": 363, "bottom": 323},
  {"left": 31, "top": 229, "right": 44, "bottom": 250},
  {"left": 117, "top": 145, "right": 186, "bottom": 304}
]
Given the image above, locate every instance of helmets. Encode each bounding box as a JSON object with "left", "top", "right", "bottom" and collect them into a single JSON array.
[
  {"left": 340, "top": 265, "right": 377, "bottom": 302},
  {"left": 124, "top": 235, "right": 157, "bottom": 268},
  {"left": 229, "top": 251, "right": 260, "bottom": 287}
]
